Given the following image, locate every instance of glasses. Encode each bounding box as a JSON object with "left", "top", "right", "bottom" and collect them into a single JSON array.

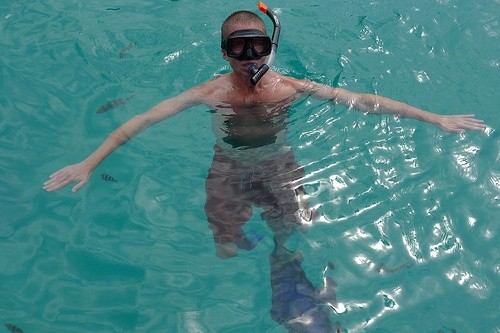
[{"left": 222, "top": 29, "right": 272, "bottom": 59}]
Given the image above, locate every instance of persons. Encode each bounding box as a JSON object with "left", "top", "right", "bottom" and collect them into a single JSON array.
[{"left": 43, "top": 11, "right": 491, "bottom": 260}]
